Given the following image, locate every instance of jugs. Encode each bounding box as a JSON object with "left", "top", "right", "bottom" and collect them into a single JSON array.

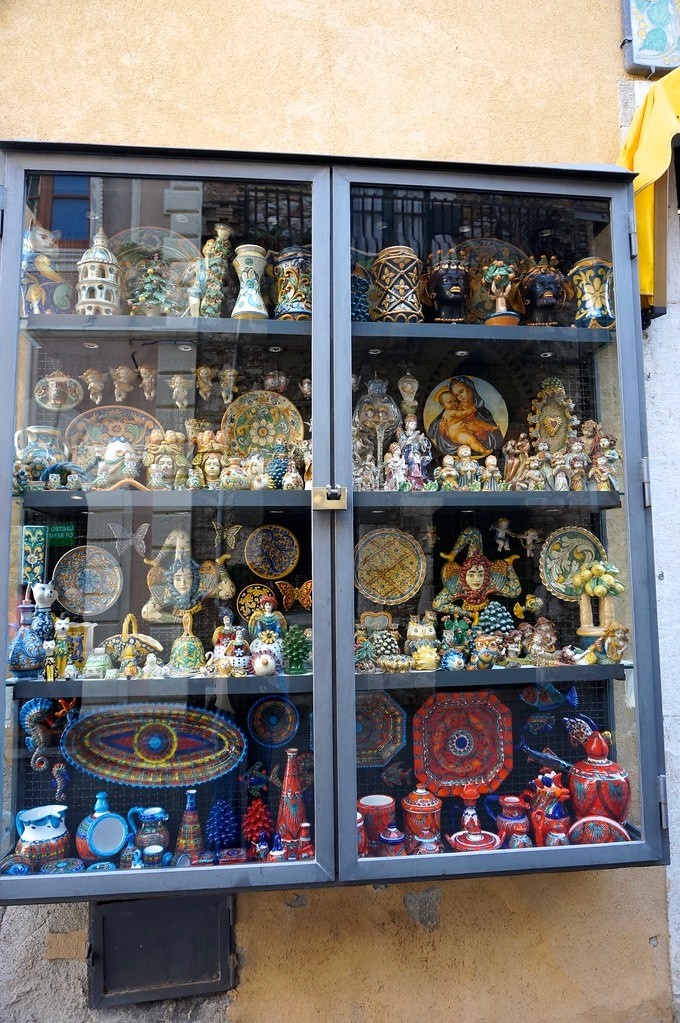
[
  {"left": 127, "top": 806, "right": 170, "bottom": 851},
  {"left": 485, "top": 796, "right": 530, "bottom": 849},
  {"left": 14, "top": 425, "right": 67, "bottom": 467},
  {"left": 15, "top": 805, "right": 72, "bottom": 871}
]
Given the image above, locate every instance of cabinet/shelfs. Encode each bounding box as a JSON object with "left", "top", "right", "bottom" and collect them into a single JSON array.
[{"left": 1, "top": 158, "right": 651, "bottom": 894}]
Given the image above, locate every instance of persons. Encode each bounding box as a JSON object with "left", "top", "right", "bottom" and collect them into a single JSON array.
[
  {"left": 142, "top": 516, "right": 631, "bottom": 679},
  {"left": 179, "top": 240, "right": 216, "bottom": 317},
  {"left": 35, "top": 363, "right": 622, "bottom": 492}
]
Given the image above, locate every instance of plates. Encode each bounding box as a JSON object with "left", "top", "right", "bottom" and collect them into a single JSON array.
[
  {"left": 444, "top": 238, "right": 530, "bottom": 325},
  {"left": 538, "top": 525, "right": 607, "bottom": 602},
  {"left": 354, "top": 528, "right": 426, "bottom": 605},
  {"left": 65, "top": 406, "right": 164, "bottom": 474},
  {"left": 52, "top": 546, "right": 123, "bottom": 617},
  {"left": 220, "top": 390, "right": 303, "bottom": 466},
  {"left": 423, "top": 376, "right": 509, "bottom": 460},
  {"left": 109, "top": 227, "right": 204, "bottom": 316},
  {"left": 237, "top": 584, "right": 277, "bottom": 622},
  {"left": 244, "top": 525, "right": 299, "bottom": 579}
]
